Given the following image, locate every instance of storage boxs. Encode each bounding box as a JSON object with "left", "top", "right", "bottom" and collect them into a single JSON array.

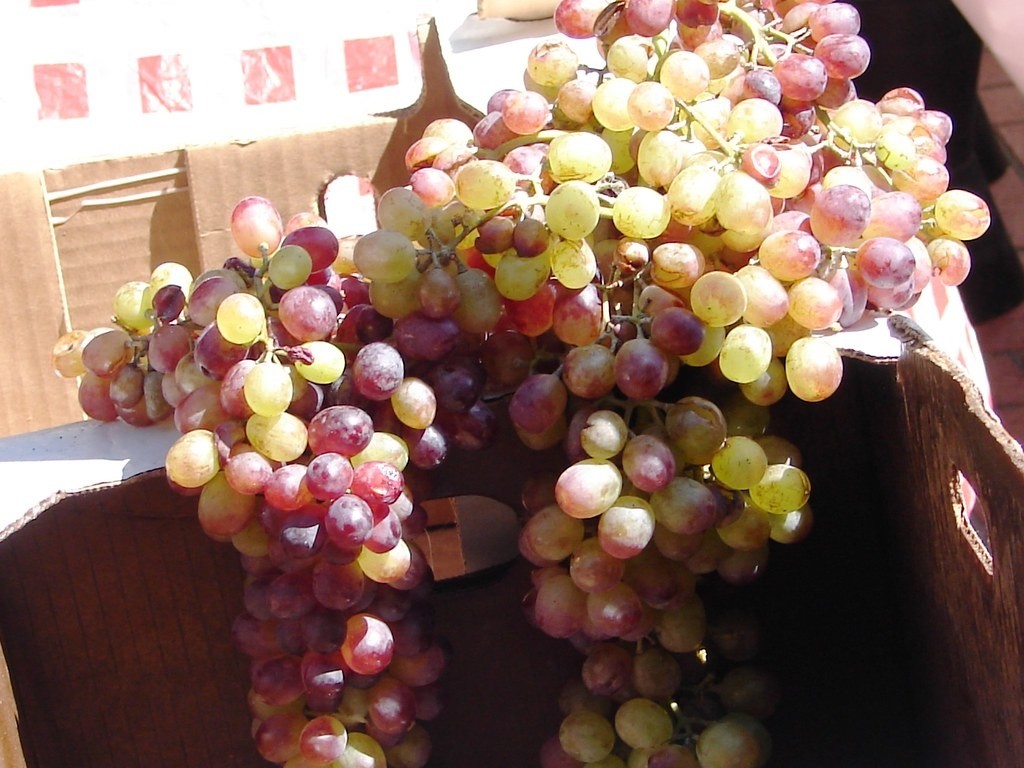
[{"left": 0, "top": 309, "right": 1023, "bottom": 767}]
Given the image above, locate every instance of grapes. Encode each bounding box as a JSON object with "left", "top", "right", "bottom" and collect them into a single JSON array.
[{"left": 54, "top": 0, "right": 991, "bottom": 768}]
